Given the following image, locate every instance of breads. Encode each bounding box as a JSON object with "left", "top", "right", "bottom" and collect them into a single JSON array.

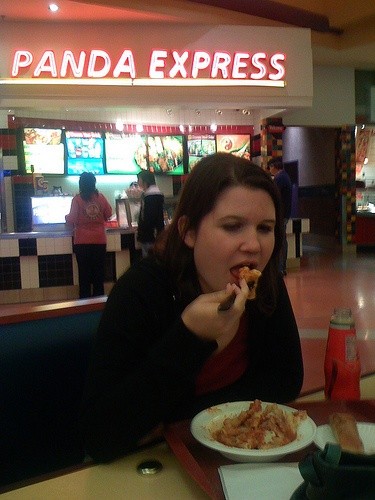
[{"left": 329, "top": 412, "right": 365, "bottom": 456}]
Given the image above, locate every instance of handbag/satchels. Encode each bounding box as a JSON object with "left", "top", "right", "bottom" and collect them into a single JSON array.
[{"left": 289, "top": 441, "right": 375, "bottom": 500}]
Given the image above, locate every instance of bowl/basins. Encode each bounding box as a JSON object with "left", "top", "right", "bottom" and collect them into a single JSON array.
[{"left": 189, "top": 400, "right": 317, "bottom": 463}]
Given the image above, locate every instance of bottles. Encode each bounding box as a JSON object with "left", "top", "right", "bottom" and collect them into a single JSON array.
[{"left": 324, "top": 305, "right": 361, "bottom": 401}]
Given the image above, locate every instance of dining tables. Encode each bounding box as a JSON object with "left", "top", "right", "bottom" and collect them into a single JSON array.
[{"left": 0, "top": 370, "right": 375, "bottom": 500}]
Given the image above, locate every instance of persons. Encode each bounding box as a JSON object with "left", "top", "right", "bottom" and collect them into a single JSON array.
[
  {"left": 134, "top": 170, "right": 164, "bottom": 257},
  {"left": 65, "top": 150, "right": 304, "bottom": 468},
  {"left": 268, "top": 156, "right": 291, "bottom": 277},
  {"left": 66, "top": 170, "right": 112, "bottom": 298}
]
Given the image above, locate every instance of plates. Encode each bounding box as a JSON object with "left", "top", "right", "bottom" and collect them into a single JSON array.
[{"left": 313, "top": 422, "right": 375, "bottom": 455}]
